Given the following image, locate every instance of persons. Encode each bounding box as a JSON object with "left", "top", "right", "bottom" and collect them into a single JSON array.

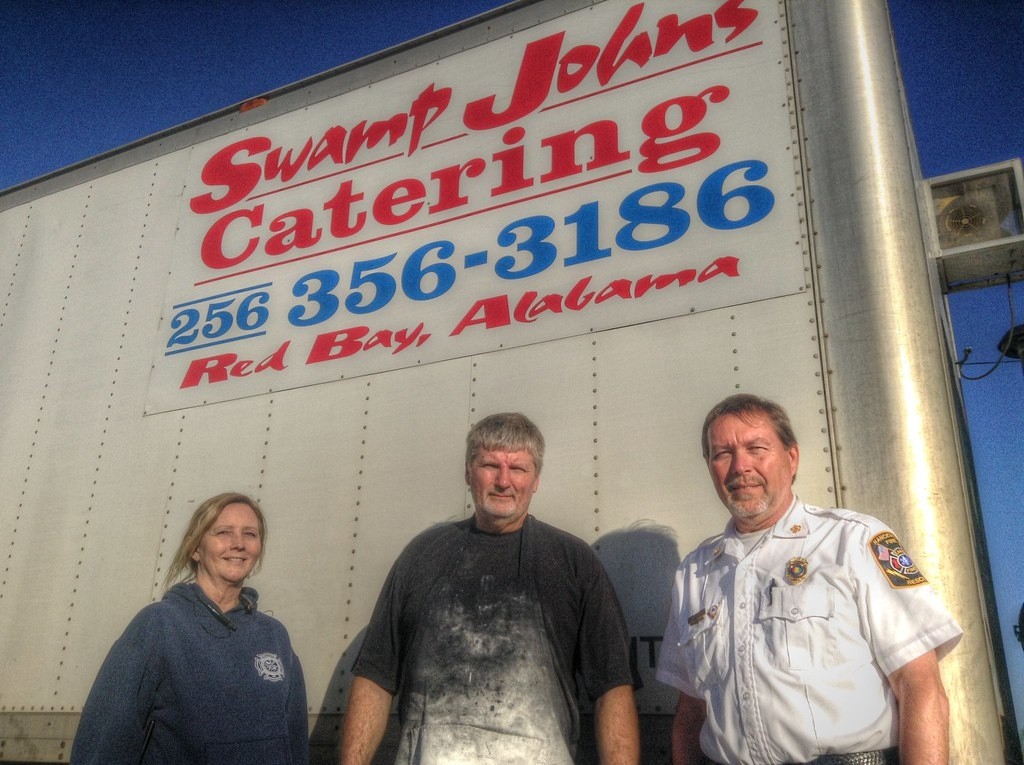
[
  {"left": 653, "top": 394, "right": 964, "bottom": 765},
  {"left": 338, "top": 414, "right": 644, "bottom": 765},
  {"left": 69, "top": 492, "right": 311, "bottom": 765}
]
